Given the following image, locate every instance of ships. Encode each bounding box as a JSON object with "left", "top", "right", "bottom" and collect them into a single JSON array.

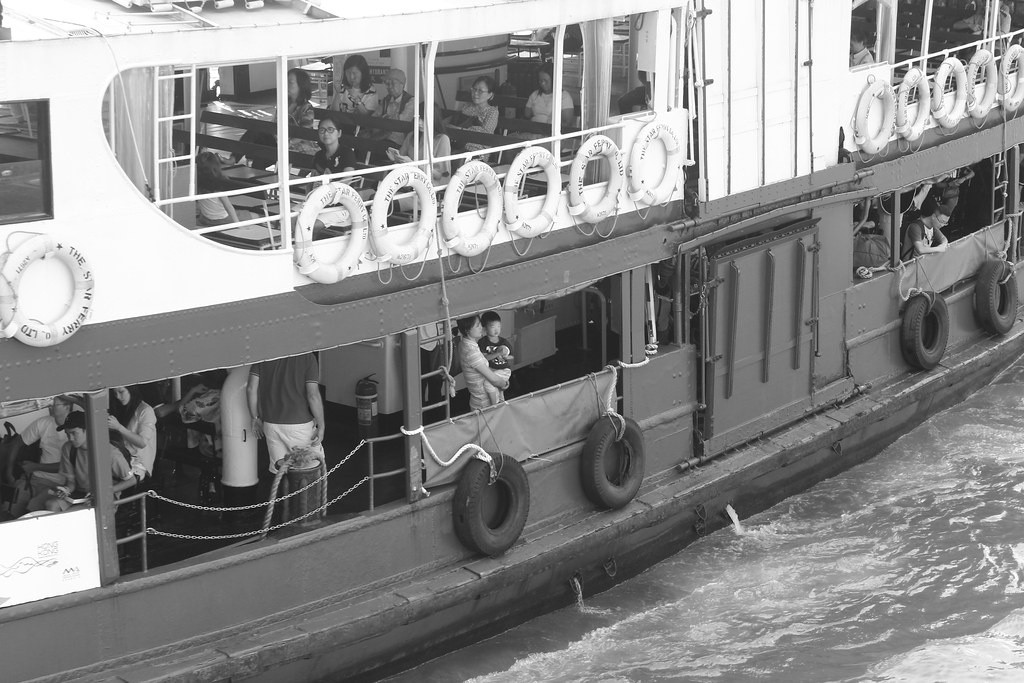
[{"left": 0, "top": 0, "right": 1024, "bottom": 683}]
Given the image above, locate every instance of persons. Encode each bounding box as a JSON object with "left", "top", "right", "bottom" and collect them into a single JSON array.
[
  {"left": 443, "top": 76, "right": 500, "bottom": 162},
  {"left": 321, "top": 55, "right": 378, "bottom": 135},
  {"left": 452, "top": 311, "right": 514, "bottom": 413},
  {"left": 352, "top": 69, "right": 413, "bottom": 162},
  {"left": 953, "top": 0, "right": 1011, "bottom": 73},
  {"left": 246, "top": 352, "right": 326, "bottom": 474},
  {"left": 196, "top": 152, "right": 251, "bottom": 225},
  {"left": 856, "top": 167, "right": 976, "bottom": 262},
  {"left": 290, "top": 116, "right": 356, "bottom": 237},
  {"left": 508, "top": 61, "right": 575, "bottom": 152},
  {"left": 421, "top": 322, "right": 446, "bottom": 413},
  {"left": 385, "top": 101, "right": 451, "bottom": 192},
  {"left": 850, "top": 26, "right": 873, "bottom": 67},
  {"left": 0, "top": 385, "right": 158, "bottom": 561},
  {"left": 214, "top": 68, "right": 317, "bottom": 170}
]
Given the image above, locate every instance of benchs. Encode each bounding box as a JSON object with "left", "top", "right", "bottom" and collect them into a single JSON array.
[
  {"left": 171, "top": 14, "right": 630, "bottom": 252},
  {"left": 852, "top": 0, "right": 1024, "bottom": 90},
  {"left": 1, "top": 405, "right": 223, "bottom": 562}
]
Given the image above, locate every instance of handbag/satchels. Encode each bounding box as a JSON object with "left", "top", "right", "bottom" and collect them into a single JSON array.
[
  {"left": 446, "top": 111, "right": 482, "bottom": 149},
  {"left": 439, "top": 335, "right": 463, "bottom": 379},
  {"left": 109, "top": 439, "right": 133, "bottom": 470}
]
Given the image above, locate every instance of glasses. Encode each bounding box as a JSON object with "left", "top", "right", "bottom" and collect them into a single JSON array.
[
  {"left": 471, "top": 87, "right": 488, "bottom": 94},
  {"left": 935, "top": 215, "right": 949, "bottom": 226},
  {"left": 318, "top": 126, "right": 338, "bottom": 134}
]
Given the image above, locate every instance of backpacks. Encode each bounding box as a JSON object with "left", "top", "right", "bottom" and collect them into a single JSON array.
[{"left": 900, "top": 220, "right": 926, "bottom": 261}]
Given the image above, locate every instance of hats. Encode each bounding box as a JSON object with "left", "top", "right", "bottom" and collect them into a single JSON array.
[
  {"left": 381, "top": 69, "right": 405, "bottom": 84},
  {"left": 56, "top": 410, "right": 86, "bottom": 431}
]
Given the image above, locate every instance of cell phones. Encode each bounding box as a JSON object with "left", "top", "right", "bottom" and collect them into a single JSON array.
[{"left": 390, "top": 148, "right": 400, "bottom": 157}]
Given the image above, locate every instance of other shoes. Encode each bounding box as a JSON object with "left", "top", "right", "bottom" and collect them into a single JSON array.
[{"left": 215, "top": 153, "right": 235, "bottom": 165}]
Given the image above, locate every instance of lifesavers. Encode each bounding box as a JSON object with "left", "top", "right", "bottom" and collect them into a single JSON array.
[
  {"left": 966, "top": 48, "right": 998, "bottom": 121},
  {"left": 296, "top": 180, "right": 370, "bottom": 284},
  {"left": 934, "top": 56, "right": 968, "bottom": 130},
  {"left": 999, "top": 43, "right": 1024, "bottom": 112},
  {"left": 854, "top": 78, "right": 897, "bottom": 155},
  {"left": 580, "top": 414, "right": 646, "bottom": 510},
  {"left": 502, "top": 145, "right": 562, "bottom": 240},
  {"left": 369, "top": 165, "right": 439, "bottom": 265},
  {"left": 0, "top": 234, "right": 96, "bottom": 350},
  {"left": 569, "top": 134, "right": 625, "bottom": 224},
  {"left": 900, "top": 291, "right": 952, "bottom": 372},
  {"left": 896, "top": 68, "right": 931, "bottom": 143},
  {"left": 453, "top": 451, "right": 531, "bottom": 558},
  {"left": 975, "top": 260, "right": 1019, "bottom": 336},
  {"left": 629, "top": 120, "right": 681, "bottom": 206},
  {"left": 443, "top": 160, "right": 503, "bottom": 257}
]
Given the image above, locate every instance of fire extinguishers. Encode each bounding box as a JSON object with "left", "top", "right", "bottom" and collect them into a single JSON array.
[{"left": 356, "top": 373, "right": 379, "bottom": 440}]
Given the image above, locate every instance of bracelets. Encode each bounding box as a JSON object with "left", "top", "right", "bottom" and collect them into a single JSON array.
[{"left": 252, "top": 416, "right": 259, "bottom": 420}]
